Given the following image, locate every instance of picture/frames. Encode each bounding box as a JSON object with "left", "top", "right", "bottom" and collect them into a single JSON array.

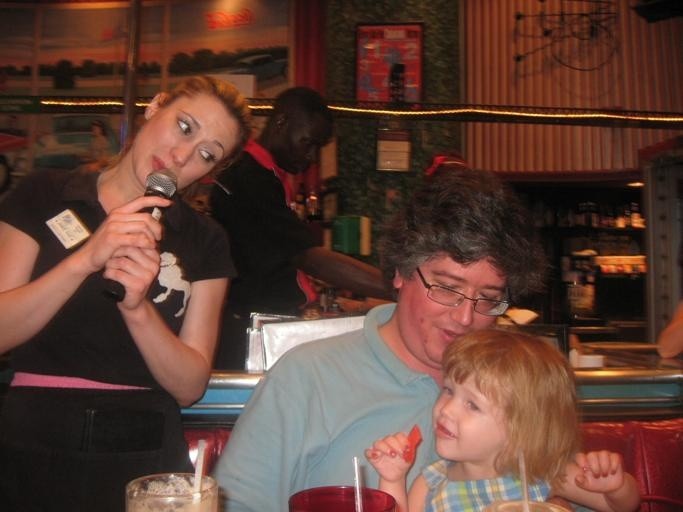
[
  {"left": 319, "top": 187, "right": 342, "bottom": 228},
  {"left": 354, "top": 23, "right": 424, "bottom": 120}
]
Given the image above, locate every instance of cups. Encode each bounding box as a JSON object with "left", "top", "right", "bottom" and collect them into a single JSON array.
[
  {"left": 125, "top": 472, "right": 219, "bottom": 512},
  {"left": 288, "top": 485, "right": 397, "bottom": 512}
]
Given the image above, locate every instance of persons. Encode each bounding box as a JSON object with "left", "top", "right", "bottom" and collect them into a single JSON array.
[
  {"left": 87, "top": 116, "right": 113, "bottom": 159},
  {"left": 207, "top": 84, "right": 399, "bottom": 372},
  {"left": 358, "top": 325, "right": 642, "bottom": 512},
  {"left": 208, "top": 160, "right": 549, "bottom": 511},
  {"left": 0, "top": 74, "right": 259, "bottom": 512},
  {"left": 652, "top": 290, "right": 682, "bottom": 358}
]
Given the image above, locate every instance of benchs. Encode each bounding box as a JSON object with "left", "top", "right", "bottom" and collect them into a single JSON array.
[{"left": 184, "top": 406, "right": 683, "bottom": 512}]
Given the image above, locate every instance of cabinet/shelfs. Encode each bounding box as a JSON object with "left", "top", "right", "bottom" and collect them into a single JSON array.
[{"left": 535, "top": 222, "right": 652, "bottom": 344}]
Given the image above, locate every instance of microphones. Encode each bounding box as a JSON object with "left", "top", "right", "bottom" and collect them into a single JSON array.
[{"left": 102, "top": 168, "right": 178, "bottom": 302}]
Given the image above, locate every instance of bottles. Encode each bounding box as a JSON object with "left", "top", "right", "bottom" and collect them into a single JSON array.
[
  {"left": 377, "top": 62, "right": 412, "bottom": 174},
  {"left": 294, "top": 180, "right": 319, "bottom": 224},
  {"left": 508, "top": 198, "right": 648, "bottom": 320}
]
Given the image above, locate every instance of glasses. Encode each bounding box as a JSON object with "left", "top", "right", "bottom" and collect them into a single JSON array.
[{"left": 414, "top": 266, "right": 513, "bottom": 321}]
